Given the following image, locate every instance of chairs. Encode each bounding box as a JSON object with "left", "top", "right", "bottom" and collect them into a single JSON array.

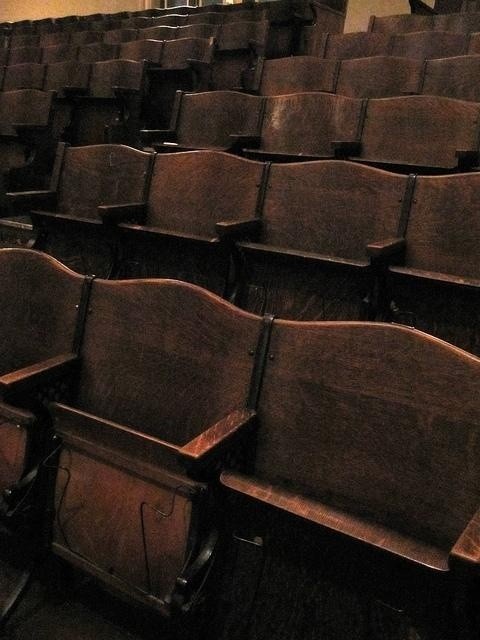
[
  {"left": 2, "top": 1, "right": 479, "bottom": 220},
  {"left": 215, "top": 158, "right": 418, "bottom": 323},
  {"left": 365, "top": 171, "right": 478, "bottom": 358},
  {"left": 5, "top": 141, "right": 159, "bottom": 279},
  {"left": 176, "top": 312, "right": 479, "bottom": 638},
  {"left": 1, "top": 248, "right": 93, "bottom": 631},
  {"left": 98, "top": 149, "right": 271, "bottom": 307},
  {"left": 2, "top": 278, "right": 273, "bottom": 636}
]
